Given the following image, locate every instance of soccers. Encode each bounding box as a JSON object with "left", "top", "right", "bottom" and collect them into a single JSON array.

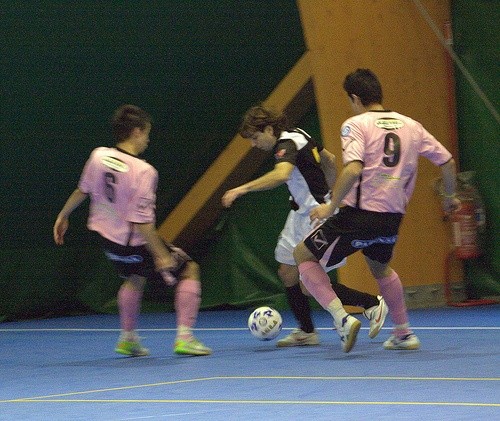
[{"left": 247, "top": 305, "right": 283, "bottom": 342}]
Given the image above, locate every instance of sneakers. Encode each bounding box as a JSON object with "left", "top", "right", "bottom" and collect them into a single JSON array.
[
  {"left": 173, "top": 334, "right": 213, "bottom": 355},
  {"left": 115, "top": 338, "right": 150, "bottom": 358},
  {"left": 333, "top": 313, "right": 362, "bottom": 352},
  {"left": 363, "top": 295, "right": 390, "bottom": 339},
  {"left": 278, "top": 327, "right": 320, "bottom": 347},
  {"left": 381, "top": 331, "right": 420, "bottom": 350}
]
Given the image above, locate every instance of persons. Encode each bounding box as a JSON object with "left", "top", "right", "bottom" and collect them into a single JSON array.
[
  {"left": 53, "top": 104, "right": 211, "bottom": 357},
  {"left": 222, "top": 106, "right": 389, "bottom": 347},
  {"left": 292, "top": 68, "right": 457, "bottom": 353},
  {"left": 456, "top": 173, "right": 486, "bottom": 299}
]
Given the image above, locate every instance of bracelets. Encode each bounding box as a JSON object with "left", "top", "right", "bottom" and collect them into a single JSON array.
[{"left": 440, "top": 190, "right": 456, "bottom": 197}]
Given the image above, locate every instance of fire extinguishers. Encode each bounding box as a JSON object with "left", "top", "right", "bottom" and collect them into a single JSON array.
[{"left": 450, "top": 182, "right": 479, "bottom": 259}]
